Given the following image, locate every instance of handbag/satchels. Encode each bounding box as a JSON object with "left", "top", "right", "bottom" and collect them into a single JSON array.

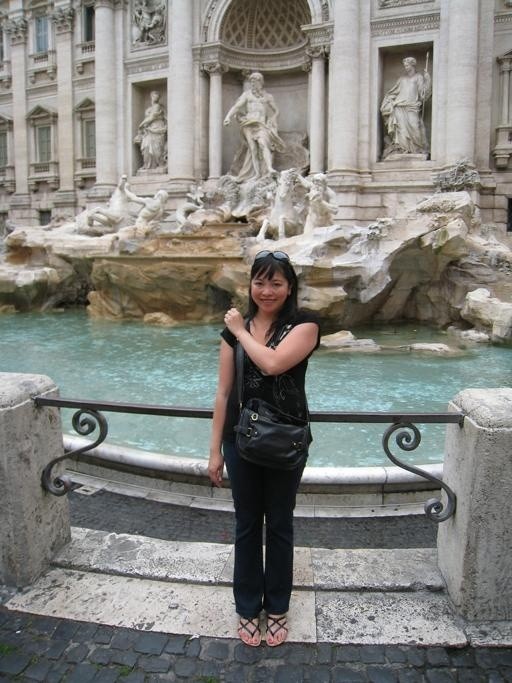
[{"left": 234, "top": 399, "right": 309, "bottom": 471}]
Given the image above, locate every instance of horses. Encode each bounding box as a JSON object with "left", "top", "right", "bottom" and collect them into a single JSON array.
[
  {"left": 87, "top": 175, "right": 139, "bottom": 234},
  {"left": 255, "top": 168, "right": 307, "bottom": 242}
]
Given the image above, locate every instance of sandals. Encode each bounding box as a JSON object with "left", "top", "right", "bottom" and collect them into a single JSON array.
[
  {"left": 266, "top": 615, "right": 287, "bottom": 647},
  {"left": 238, "top": 616, "right": 262, "bottom": 647}
]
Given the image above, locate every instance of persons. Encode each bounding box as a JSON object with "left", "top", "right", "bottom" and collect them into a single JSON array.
[
  {"left": 381, "top": 57, "right": 433, "bottom": 154},
  {"left": 133, "top": 91, "right": 169, "bottom": 170},
  {"left": 144, "top": 6, "right": 164, "bottom": 42},
  {"left": 222, "top": 70, "right": 289, "bottom": 183},
  {"left": 205, "top": 250, "right": 321, "bottom": 647},
  {"left": 132, "top": 1, "right": 158, "bottom": 44}
]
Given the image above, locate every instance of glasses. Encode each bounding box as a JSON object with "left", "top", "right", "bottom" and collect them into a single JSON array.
[{"left": 255, "top": 250, "right": 290, "bottom": 265}]
